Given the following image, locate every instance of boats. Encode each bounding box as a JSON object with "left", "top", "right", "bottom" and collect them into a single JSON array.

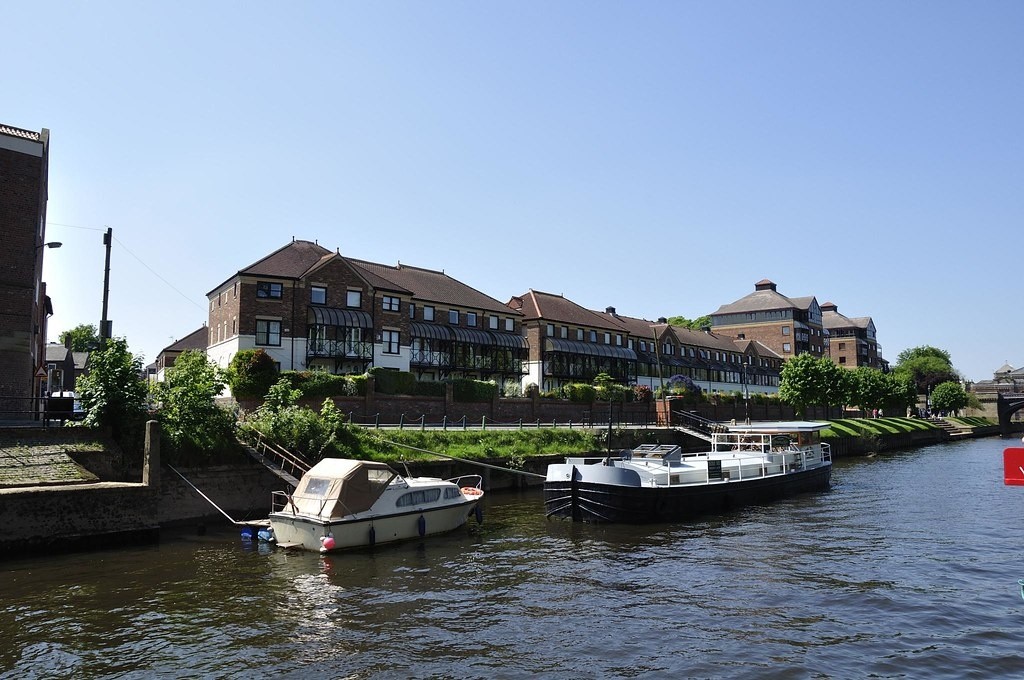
[
  {"left": 543, "top": 396, "right": 833, "bottom": 527},
  {"left": 268, "top": 458, "right": 485, "bottom": 554}
]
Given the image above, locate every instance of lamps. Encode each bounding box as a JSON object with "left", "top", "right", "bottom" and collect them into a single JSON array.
[{"left": 36, "top": 242, "right": 62, "bottom": 249}]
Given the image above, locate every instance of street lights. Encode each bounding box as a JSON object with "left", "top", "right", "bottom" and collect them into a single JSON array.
[{"left": 744, "top": 362, "right": 750, "bottom": 425}]
